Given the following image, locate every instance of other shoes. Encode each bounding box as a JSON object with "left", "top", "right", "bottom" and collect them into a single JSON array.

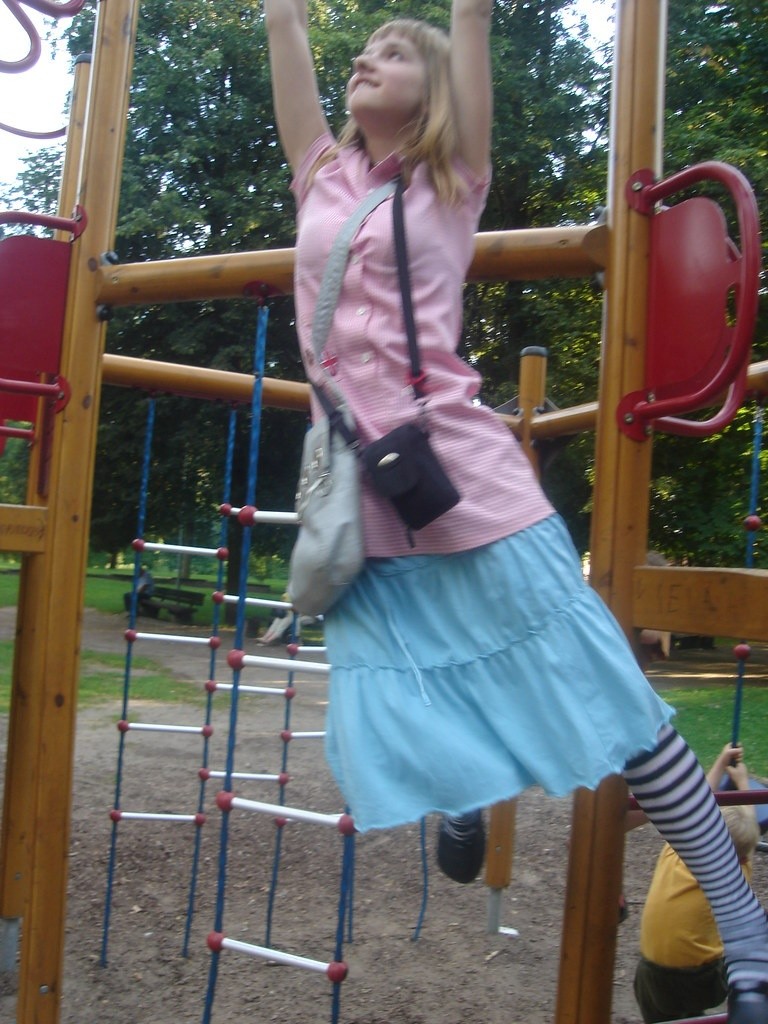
[
  {"left": 725, "top": 979, "right": 768, "bottom": 1023},
  {"left": 439, "top": 813, "right": 484, "bottom": 882}
]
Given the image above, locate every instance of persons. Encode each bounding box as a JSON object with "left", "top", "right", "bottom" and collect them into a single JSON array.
[
  {"left": 634, "top": 741, "right": 761, "bottom": 1023},
  {"left": 261, "top": 1, "right": 768, "bottom": 1024}
]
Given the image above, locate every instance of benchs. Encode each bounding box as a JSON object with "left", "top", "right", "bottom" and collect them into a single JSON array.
[{"left": 136, "top": 586, "right": 204, "bottom": 624}]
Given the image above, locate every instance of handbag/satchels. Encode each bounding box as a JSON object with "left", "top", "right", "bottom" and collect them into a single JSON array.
[
  {"left": 358, "top": 422, "right": 460, "bottom": 530},
  {"left": 288, "top": 403, "right": 369, "bottom": 618}
]
